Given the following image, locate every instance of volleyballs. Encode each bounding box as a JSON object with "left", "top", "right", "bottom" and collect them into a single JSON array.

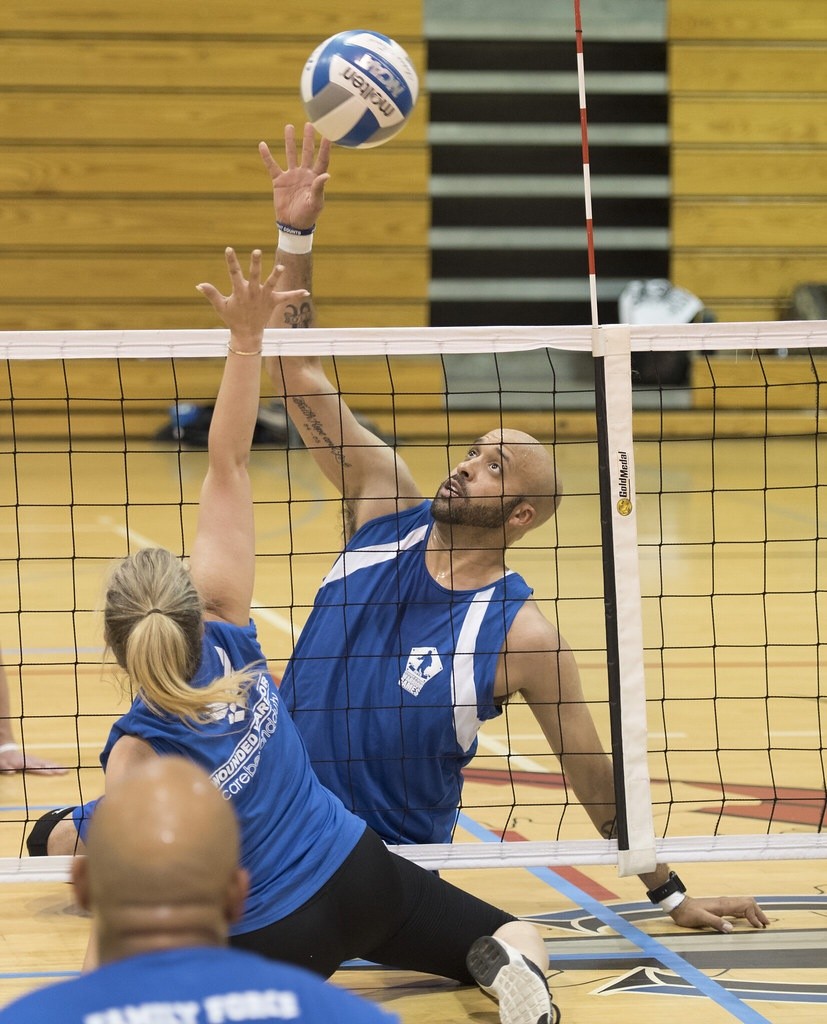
[{"left": 297, "top": 25, "right": 422, "bottom": 152}]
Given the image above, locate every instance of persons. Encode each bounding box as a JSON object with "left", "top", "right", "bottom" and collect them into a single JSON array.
[
  {"left": 101, "top": 244, "right": 558, "bottom": 1024},
  {"left": 0, "top": 754, "right": 399, "bottom": 1024},
  {"left": 24, "top": 119, "right": 771, "bottom": 935}
]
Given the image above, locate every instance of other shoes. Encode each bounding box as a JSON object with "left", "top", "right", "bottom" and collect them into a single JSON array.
[{"left": 466, "top": 936, "right": 561, "bottom": 1024}]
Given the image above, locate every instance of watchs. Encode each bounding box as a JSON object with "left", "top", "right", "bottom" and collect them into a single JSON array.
[{"left": 646, "top": 871, "right": 686, "bottom": 905}]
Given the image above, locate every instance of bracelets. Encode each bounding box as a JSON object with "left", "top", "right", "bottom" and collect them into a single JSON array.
[
  {"left": 227, "top": 341, "right": 264, "bottom": 356},
  {"left": 659, "top": 891, "right": 685, "bottom": 914},
  {"left": 274, "top": 219, "right": 315, "bottom": 236},
  {"left": 275, "top": 231, "right": 313, "bottom": 255}
]
